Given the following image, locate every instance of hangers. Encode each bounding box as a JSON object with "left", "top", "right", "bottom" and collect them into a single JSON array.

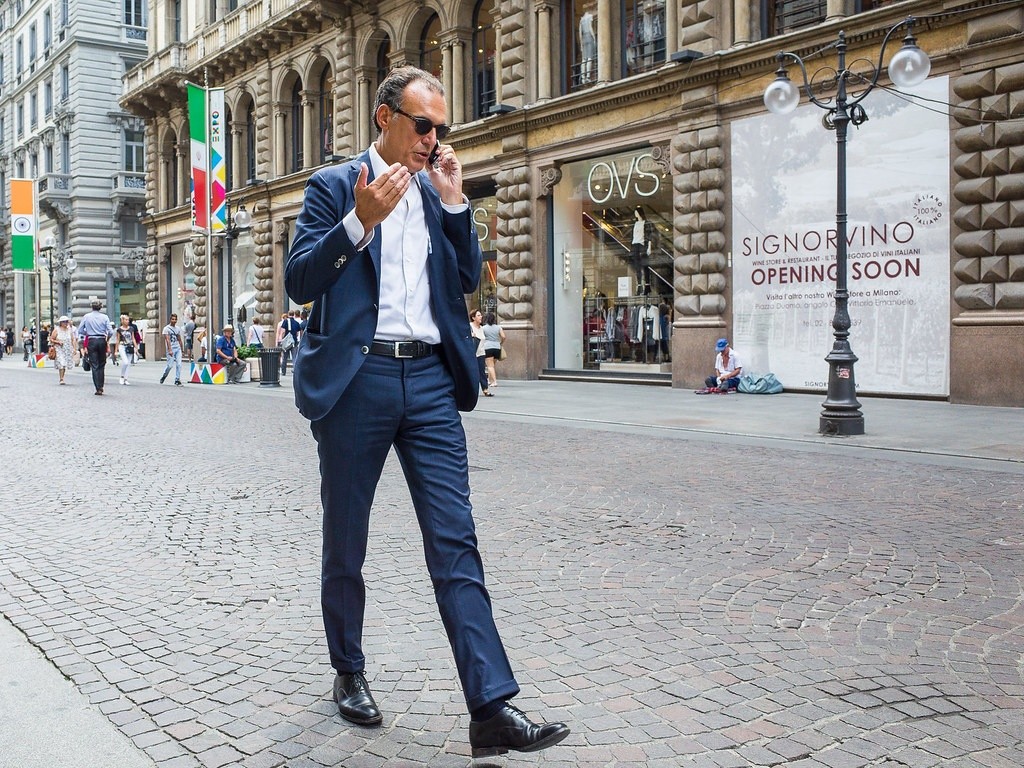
[{"left": 633, "top": 297, "right": 651, "bottom": 312}]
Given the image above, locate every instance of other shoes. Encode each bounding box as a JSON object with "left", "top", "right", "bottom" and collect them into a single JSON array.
[
  {"left": 160, "top": 378, "right": 164, "bottom": 383},
  {"left": 228, "top": 379, "right": 239, "bottom": 384},
  {"left": 60, "top": 381, "right": 65, "bottom": 385},
  {"left": 484, "top": 392, "right": 494, "bottom": 396},
  {"left": 95, "top": 387, "right": 103, "bottom": 395},
  {"left": 119, "top": 378, "right": 131, "bottom": 385},
  {"left": 488, "top": 382, "right": 497, "bottom": 387},
  {"left": 174, "top": 381, "right": 183, "bottom": 387}
]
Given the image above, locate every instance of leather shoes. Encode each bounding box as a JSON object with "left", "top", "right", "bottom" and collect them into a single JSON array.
[
  {"left": 469, "top": 702, "right": 571, "bottom": 758},
  {"left": 333, "top": 669, "right": 383, "bottom": 724}
]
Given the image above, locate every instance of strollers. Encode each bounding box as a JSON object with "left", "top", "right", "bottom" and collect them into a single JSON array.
[{"left": 23, "top": 337, "right": 34, "bottom": 361}]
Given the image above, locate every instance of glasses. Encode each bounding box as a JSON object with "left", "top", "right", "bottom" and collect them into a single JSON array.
[{"left": 382, "top": 102, "right": 451, "bottom": 140}]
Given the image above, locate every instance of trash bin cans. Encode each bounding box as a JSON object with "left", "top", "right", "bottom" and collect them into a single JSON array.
[{"left": 256, "top": 347, "right": 283, "bottom": 389}]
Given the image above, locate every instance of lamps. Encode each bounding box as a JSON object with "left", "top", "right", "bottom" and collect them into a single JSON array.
[
  {"left": 488, "top": 104, "right": 516, "bottom": 113},
  {"left": 246, "top": 179, "right": 263, "bottom": 186},
  {"left": 325, "top": 154, "right": 345, "bottom": 163},
  {"left": 670, "top": 48, "right": 703, "bottom": 62}
]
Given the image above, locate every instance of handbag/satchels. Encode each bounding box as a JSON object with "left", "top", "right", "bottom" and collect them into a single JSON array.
[
  {"left": 49, "top": 346, "right": 56, "bottom": 359},
  {"left": 499, "top": 343, "right": 507, "bottom": 361},
  {"left": 82, "top": 353, "right": 90, "bottom": 371},
  {"left": 737, "top": 372, "right": 783, "bottom": 393},
  {"left": 282, "top": 332, "right": 295, "bottom": 351},
  {"left": 259, "top": 343, "right": 264, "bottom": 348}
]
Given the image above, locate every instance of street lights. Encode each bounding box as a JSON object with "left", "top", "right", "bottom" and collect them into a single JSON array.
[
  {"left": 223, "top": 193, "right": 252, "bottom": 337},
  {"left": 40, "top": 236, "right": 78, "bottom": 332},
  {"left": 763, "top": 15, "right": 931, "bottom": 439}
]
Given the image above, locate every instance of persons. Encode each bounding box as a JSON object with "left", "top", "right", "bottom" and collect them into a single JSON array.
[
  {"left": 247, "top": 317, "right": 265, "bottom": 348},
  {"left": 184, "top": 299, "right": 195, "bottom": 324},
  {"left": 184, "top": 315, "right": 196, "bottom": 363},
  {"left": 160, "top": 313, "right": 184, "bottom": 387},
  {"left": 469, "top": 309, "right": 495, "bottom": 397},
  {"left": 78, "top": 299, "right": 113, "bottom": 395},
  {"left": 705, "top": 338, "right": 743, "bottom": 390},
  {"left": 71, "top": 317, "right": 141, "bottom": 368},
  {"left": 21, "top": 319, "right": 50, "bottom": 362},
  {"left": 237, "top": 304, "right": 247, "bottom": 347},
  {"left": 631, "top": 206, "right": 652, "bottom": 296},
  {"left": 579, "top": 11, "right": 595, "bottom": 85},
  {"left": 0, "top": 327, "right": 16, "bottom": 360},
  {"left": 482, "top": 312, "right": 505, "bottom": 386},
  {"left": 216, "top": 324, "right": 246, "bottom": 385},
  {"left": 49, "top": 316, "right": 77, "bottom": 385},
  {"left": 276, "top": 309, "right": 308, "bottom": 376},
  {"left": 283, "top": 65, "right": 572, "bottom": 758},
  {"left": 115, "top": 314, "right": 138, "bottom": 386},
  {"left": 198, "top": 328, "right": 220, "bottom": 362}
]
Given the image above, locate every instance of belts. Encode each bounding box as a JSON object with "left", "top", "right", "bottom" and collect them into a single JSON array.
[{"left": 367, "top": 342, "right": 442, "bottom": 359}]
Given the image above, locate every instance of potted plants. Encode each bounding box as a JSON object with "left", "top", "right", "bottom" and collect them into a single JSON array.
[{"left": 235, "top": 343, "right": 263, "bottom": 382}]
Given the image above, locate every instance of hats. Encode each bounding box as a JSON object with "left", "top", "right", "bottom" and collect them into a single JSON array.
[
  {"left": 715, "top": 339, "right": 727, "bottom": 351},
  {"left": 57, "top": 316, "right": 70, "bottom": 323},
  {"left": 222, "top": 324, "right": 235, "bottom": 332}
]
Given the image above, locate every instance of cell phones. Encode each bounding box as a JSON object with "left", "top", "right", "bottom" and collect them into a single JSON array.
[{"left": 427, "top": 140, "right": 441, "bottom": 165}]
